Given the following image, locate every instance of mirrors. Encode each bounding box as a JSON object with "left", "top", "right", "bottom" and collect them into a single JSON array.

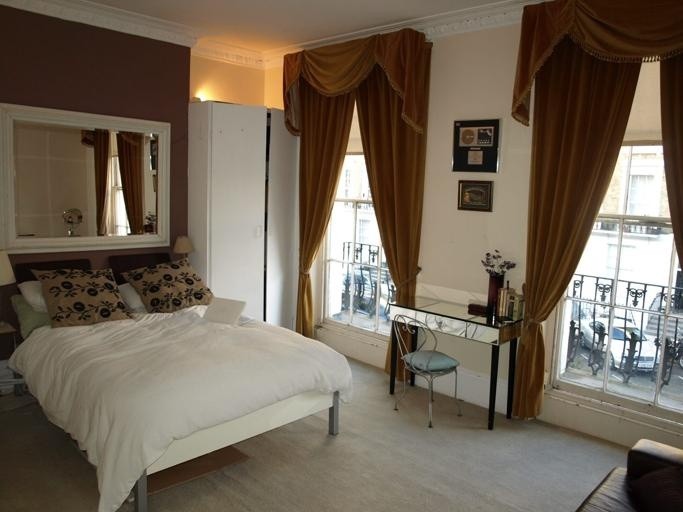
[{"left": 0, "top": 103, "right": 171, "bottom": 250}]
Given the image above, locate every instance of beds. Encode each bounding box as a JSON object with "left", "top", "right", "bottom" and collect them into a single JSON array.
[{"left": 7, "top": 246, "right": 354, "bottom": 511}]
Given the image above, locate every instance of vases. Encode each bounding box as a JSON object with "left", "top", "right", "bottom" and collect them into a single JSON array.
[{"left": 487, "top": 275, "right": 504, "bottom": 317}]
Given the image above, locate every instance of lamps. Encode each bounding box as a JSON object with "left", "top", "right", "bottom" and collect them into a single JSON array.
[
  {"left": 173, "top": 236, "right": 194, "bottom": 258},
  {"left": 0, "top": 251, "right": 16, "bottom": 287}
]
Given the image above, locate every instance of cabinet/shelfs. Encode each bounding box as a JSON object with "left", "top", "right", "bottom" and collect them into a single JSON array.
[{"left": 188, "top": 99, "right": 300, "bottom": 332}]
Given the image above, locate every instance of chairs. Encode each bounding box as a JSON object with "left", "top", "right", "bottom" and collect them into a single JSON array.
[{"left": 393, "top": 314, "right": 463, "bottom": 428}]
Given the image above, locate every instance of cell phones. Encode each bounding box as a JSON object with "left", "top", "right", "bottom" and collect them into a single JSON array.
[{"left": 496, "top": 316, "right": 515, "bottom": 323}]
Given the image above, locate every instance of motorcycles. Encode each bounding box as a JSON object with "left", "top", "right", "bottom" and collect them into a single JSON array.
[{"left": 655, "top": 327, "right": 682, "bottom": 370}]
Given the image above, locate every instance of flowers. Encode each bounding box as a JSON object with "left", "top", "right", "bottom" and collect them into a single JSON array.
[{"left": 481, "top": 249, "right": 516, "bottom": 278}]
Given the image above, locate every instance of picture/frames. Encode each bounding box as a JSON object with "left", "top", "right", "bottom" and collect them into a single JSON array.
[
  {"left": 457, "top": 179, "right": 493, "bottom": 212},
  {"left": 451, "top": 118, "right": 502, "bottom": 173}
]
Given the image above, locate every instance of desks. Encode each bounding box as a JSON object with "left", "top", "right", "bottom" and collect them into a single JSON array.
[{"left": 389, "top": 296, "right": 523, "bottom": 430}]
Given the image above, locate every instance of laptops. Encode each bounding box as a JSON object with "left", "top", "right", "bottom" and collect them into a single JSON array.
[{"left": 202, "top": 297, "right": 253, "bottom": 328}]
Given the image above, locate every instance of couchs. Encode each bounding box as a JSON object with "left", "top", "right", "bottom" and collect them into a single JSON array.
[{"left": 575, "top": 439, "right": 683, "bottom": 511}]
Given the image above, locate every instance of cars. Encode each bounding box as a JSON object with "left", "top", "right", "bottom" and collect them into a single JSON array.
[
  {"left": 574, "top": 314, "right": 661, "bottom": 376},
  {"left": 339, "top": 269, "right": 391, "bottom": 322},
  {"left": 571, "top": 302, "right": 589, "bottom": 344}
]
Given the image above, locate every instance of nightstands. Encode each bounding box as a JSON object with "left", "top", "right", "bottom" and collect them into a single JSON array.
[{"left": 0, "top": 321, "right": 23, "bottom": 395}]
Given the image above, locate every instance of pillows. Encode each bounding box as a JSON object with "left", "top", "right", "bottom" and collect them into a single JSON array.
[
  {"left": 121, "top": 258, "right": 213, "bottom": 314},
  {"left": 31, "top": 268, "right": 135, "bottom": 328},
  {"left": 117, "top": 282, "right": 144, "bottom": 308},
  {"left": 10, "top": 295, "right": 52, "bottom": 339},
  {"left": 17, "top": 281, "right": 48, "bottom": 314}
]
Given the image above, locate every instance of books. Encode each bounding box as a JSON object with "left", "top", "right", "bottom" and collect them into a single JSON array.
[{"left": 495, "top": 287, "right": 524, "bottom": 322}]
